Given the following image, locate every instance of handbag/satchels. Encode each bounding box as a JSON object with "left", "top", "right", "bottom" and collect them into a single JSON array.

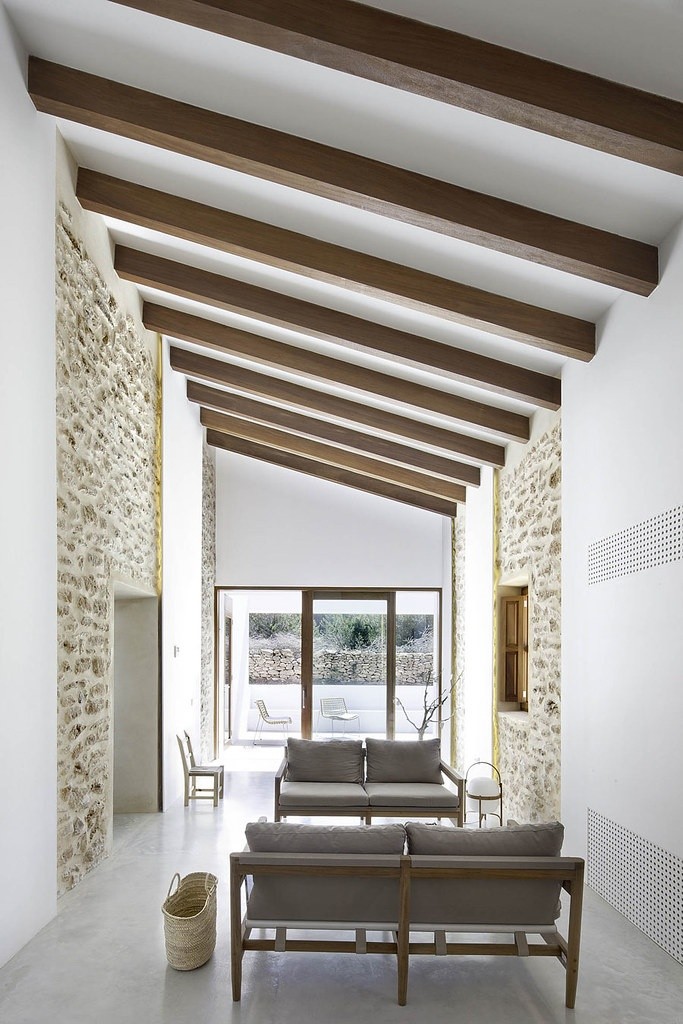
[{"left": 162, "top": 872, "right": 218, "bottom": 971}]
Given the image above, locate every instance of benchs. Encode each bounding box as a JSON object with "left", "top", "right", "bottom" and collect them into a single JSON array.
[
  {"left": 274, "top": 737, "right": 464, "bottom": 828},
  {"left": 230, "top": 817, "right": 585, "bottom": 1009}
]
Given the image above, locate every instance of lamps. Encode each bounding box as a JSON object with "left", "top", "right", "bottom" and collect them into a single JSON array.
[{"left": 465, "top": 762, "right": 502, "bottom": 829}]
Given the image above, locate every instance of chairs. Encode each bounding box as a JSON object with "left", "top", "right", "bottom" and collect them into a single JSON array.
[
  {"left": 176, "top": 730, "right": 225, "bottom": 807},
  {"left": 317, "top": 698, "right": 360, "bottom": 737},
  {"left": 253, "top": 700, "right": 292, "bottom": 743}
]
